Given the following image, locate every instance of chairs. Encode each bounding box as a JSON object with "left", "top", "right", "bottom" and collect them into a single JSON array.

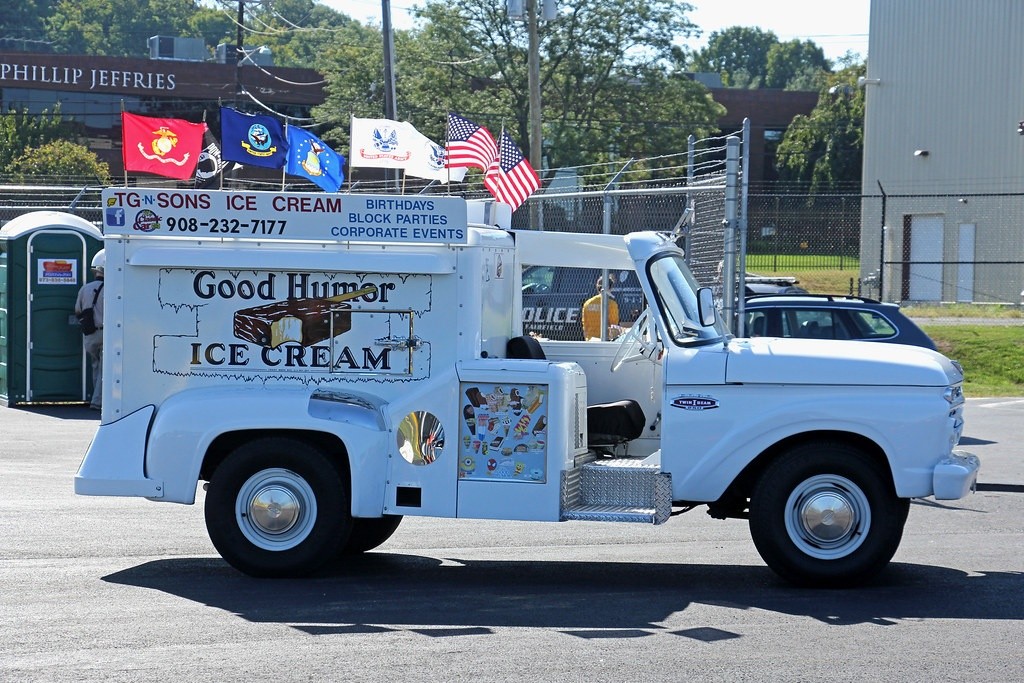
[{"left": 505, "top": 335, "right": 645, "bottom": 458}]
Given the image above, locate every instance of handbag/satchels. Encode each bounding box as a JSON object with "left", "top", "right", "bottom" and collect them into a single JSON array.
[{"left": 77, "top": 307, "right": 97, "bottom": 336}]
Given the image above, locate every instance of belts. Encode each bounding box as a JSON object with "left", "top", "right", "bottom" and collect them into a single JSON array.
[{"left": 95, "top": 327, "right": 103, "bottom": 330}]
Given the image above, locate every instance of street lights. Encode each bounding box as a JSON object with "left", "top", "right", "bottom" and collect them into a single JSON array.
[{"left": 232, "top": 44, "right": 275, "bottom": 109}]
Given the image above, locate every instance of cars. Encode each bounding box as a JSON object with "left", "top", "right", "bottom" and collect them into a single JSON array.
[{"left": 734, "top": 275, "right": 812, "bottom": 298}]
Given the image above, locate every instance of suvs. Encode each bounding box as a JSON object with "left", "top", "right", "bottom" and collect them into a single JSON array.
[{"left": 723, "top": 291, "right": 940, "bottom": 351}]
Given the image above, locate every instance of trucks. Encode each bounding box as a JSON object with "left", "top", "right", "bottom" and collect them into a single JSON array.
[{"left": 73, "top": 137, "right": 982, "bottom": 594}]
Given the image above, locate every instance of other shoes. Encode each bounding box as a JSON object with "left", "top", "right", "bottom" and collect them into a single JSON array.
[{"left": 90, "top": 403, "right": 101, "bottom": 410}]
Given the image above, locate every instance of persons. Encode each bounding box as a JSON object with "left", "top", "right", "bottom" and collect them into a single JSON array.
[
  {"left": 582, "top": 276, "right": 620, "bottom": 342},
  {"left": 74, "top": 266, "right": 104, "bottom": 410}
]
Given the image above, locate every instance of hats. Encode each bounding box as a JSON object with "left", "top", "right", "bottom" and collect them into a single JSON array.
[{"left": 91, "top": 266, "right": 104, "bottom": 274}]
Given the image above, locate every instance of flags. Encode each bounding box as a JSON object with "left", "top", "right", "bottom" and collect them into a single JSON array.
[
  {"left": 349, "top": 113, "right": 417, "bottom": 170},
  {"left": 219, "top": 105, "right": 291, "bottom": 170},
  {"left": 120, "top": 110, "right": 207, "bottom": 182},
  {"left": 444, "top": 108, "right": 500, "bottom": 175},
  {"left": 283, "top": 122, "right": 347, "bottom": 193},
  {"left": 403, "top": 120, "right": 469, "bottom": 184},
  {"left": 485, "top": 117, "right": 541, "bottom": 215},
  {"left": 194, "top": 120, "right": 250, "bottom": 189}
]
